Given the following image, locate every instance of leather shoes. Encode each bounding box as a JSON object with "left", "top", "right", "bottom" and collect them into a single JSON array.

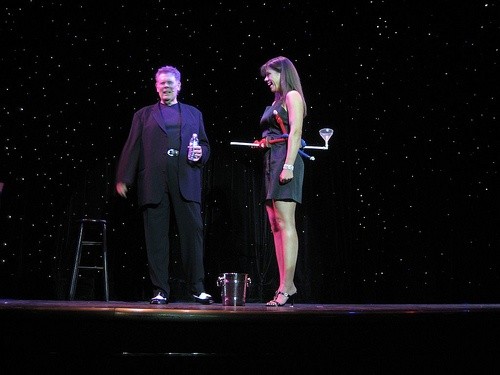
[
  {"left": 150, "top": 290, "right": 167, "bottom": 304},
  {"left": 192, "top": 291, "right": 214, "bottom": 304}
]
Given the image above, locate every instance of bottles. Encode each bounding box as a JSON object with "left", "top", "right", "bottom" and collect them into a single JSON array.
[{"left": 188, "top": 133, "right": 199, "bottom": 160}]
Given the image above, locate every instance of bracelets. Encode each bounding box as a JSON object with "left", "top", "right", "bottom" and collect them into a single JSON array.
[{"left": 283, "top": 163, "right": 294, "bottom": 170}]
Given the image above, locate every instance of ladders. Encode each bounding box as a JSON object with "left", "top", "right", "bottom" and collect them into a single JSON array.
[{"left": 69, "top": 219, "right": 109, "bottom": 303}]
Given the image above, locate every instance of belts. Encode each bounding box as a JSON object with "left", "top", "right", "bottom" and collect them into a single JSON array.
[{"left": 167, "top": 149, "right": 179, "bottom": 157}]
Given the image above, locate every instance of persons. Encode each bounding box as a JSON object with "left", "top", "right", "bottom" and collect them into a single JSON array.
[
  {"left": 258, "top": 55, "right": 308, "bottom": 307},
  {"left": 114, "top": 65, "right": 211, "bottom": 306}
]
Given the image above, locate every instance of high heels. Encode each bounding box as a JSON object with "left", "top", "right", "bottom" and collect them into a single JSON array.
[{"left": 265, "top": 290, "right": 298, "bottom": 307}]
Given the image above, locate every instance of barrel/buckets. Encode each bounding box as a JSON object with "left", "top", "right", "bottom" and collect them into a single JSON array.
[{"left": 218, "top": 273, "right": 251, "bottom": 306}]
